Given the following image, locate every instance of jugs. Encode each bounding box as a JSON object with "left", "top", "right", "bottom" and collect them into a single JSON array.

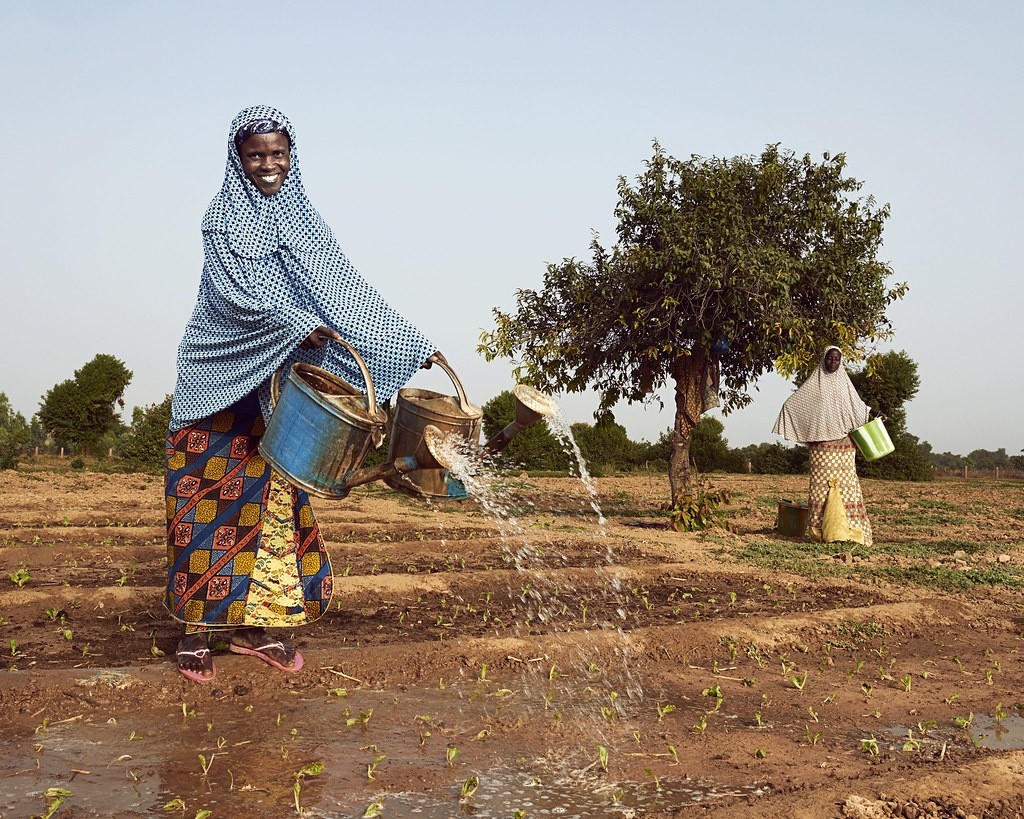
[
  {"left": 258, "top": 336, "right": 451, "bottom": 501},
  {"left": 383, "top": 356, "right": 558, "bottom": 500}
]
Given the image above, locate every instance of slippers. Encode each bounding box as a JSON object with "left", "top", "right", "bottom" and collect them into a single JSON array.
[
  {"left": 176, "top": 649, "right": 217, "bottom": 681},
  {"left": 230, "top": 640, "right": 304, "bottom": 672}
]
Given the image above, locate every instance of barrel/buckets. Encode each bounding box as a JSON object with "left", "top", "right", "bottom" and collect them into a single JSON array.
[
  {"left": 777, "top": 500, "right": 810, "bottom": 541},
  {"left": 850, "top": 413, "right": 896, "bottom": 462}
]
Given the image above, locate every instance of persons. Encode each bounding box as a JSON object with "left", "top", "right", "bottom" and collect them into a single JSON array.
[
  {"left": 165, "top": 105, "right": 448, "bottom": 682},
  {"left": 771, "top": 345, "right": 887, "bottom": 546}
]
